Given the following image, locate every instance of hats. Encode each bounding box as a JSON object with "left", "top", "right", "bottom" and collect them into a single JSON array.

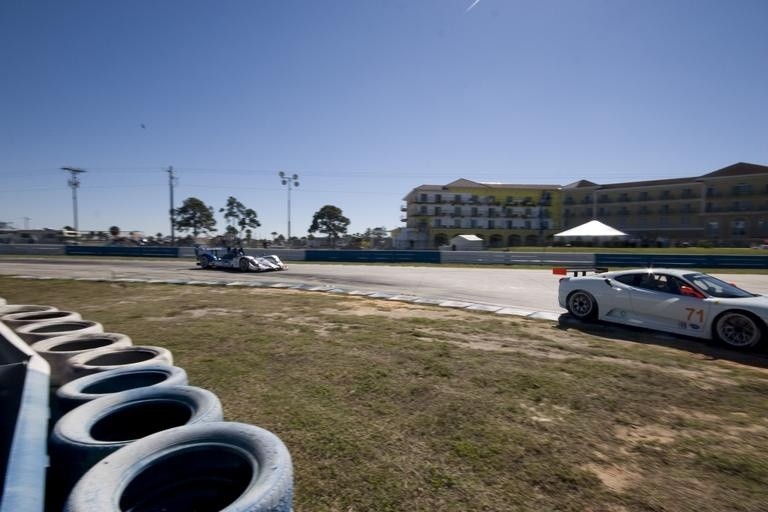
[
  {"left": 1, "top": 228, "right": 152, "bottom": 248},
  {"left": 556, "top": 266, "right": 768, "bottom": 359}
]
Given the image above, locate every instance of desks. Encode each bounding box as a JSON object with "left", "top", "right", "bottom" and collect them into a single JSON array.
[{"left": 278, "top": 170, "right": 300, "bottom": 249}]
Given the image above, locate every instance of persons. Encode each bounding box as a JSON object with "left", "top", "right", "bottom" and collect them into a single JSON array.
[{"left": 227, "top": 246, "right": 245, "bottom": 256}]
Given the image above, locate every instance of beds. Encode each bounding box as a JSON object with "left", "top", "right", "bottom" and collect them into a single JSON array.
[{"left": 552, "top": 218, "right": 630, "bottom": 238}]
